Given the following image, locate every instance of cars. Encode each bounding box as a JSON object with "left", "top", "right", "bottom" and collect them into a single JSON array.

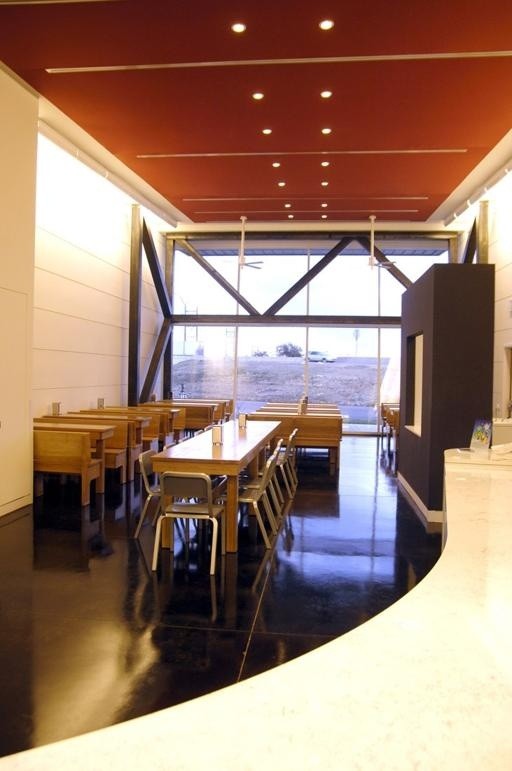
[
  {"left": 303, "top": 349, "right": 338, "bottom": 362},
  {"left": 274, "top": 342, "right": 302, "bottom": 357}
]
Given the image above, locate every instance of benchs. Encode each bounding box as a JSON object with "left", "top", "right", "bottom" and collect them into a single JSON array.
[{"left": 380, "top": 403, "right": 400, "bottom": 478}]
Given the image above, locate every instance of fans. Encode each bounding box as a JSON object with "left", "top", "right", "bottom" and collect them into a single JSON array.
[
  {"left": 238, "top": 216, "right": 263, "bottom": 270},
  {"left": 367, "top": 215, "right": 396, "bottom": 271}
]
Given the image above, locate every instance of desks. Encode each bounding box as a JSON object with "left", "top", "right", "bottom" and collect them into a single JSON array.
[{"left": 150, "top": 421, "right": 282, "bottom": 553}]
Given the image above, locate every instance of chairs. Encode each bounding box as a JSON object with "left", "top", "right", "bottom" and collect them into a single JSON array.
[{"left": 133, "top": 425, "right": 299, "bottom": 577}]
